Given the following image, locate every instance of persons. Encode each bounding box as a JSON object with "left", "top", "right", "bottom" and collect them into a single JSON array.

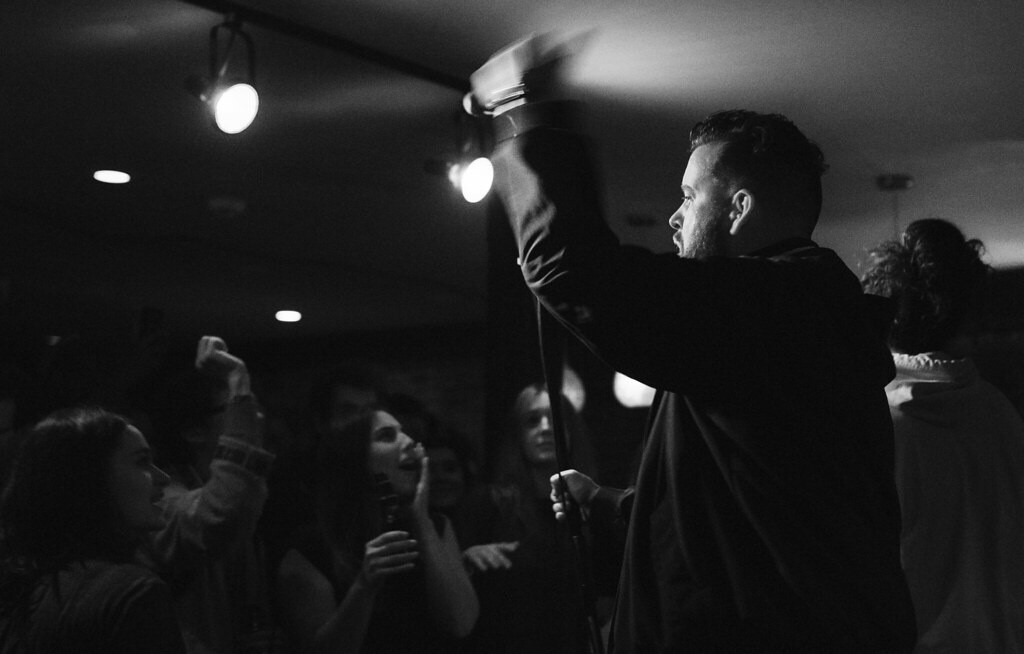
[
  {"left": 0, "top": 335, "right": 603, "bottom": 654},
  {"left": 466, "top": 32, "right": 920, "bottom": 654},
  {"left": 864, "top": 217, "right": 1024, "bottom": 653}
]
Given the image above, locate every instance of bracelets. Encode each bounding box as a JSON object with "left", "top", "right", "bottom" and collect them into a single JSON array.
[
  {"left": 222, "top": 393, "right": 251, "bottom": 405},
  {"left": 483, "top": 84, "right": 526, "bottom": 114}
]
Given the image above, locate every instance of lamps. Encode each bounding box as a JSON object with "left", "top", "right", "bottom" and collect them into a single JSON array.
[
  {"left": 421, "top": 99, "right": 494, "bottom": 202},
  {"left": 181, "top": 17, "right": 259, "bottom": 134}
]
